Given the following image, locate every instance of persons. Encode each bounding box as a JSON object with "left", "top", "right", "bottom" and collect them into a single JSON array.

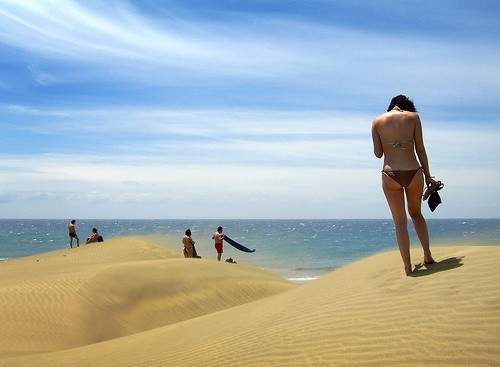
[
  {"left": 212, "top": 225, "right": 225, "bottom": 261},
  {"left": 86, "top": 228, "right": 103, "bottom": 244},
  {"left": 182, "top": 229, "right": 196, "bottom": 257},
  {"left": 68, "top": 219, "right": 80, "bottom": 248},
  {"left": 371, "top": 94, "right": 440, "bottom": 275}
]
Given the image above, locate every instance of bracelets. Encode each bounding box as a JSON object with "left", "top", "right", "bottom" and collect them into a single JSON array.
[{"left": 426, "top": 176, "right": 430, "bottom": 178}]
